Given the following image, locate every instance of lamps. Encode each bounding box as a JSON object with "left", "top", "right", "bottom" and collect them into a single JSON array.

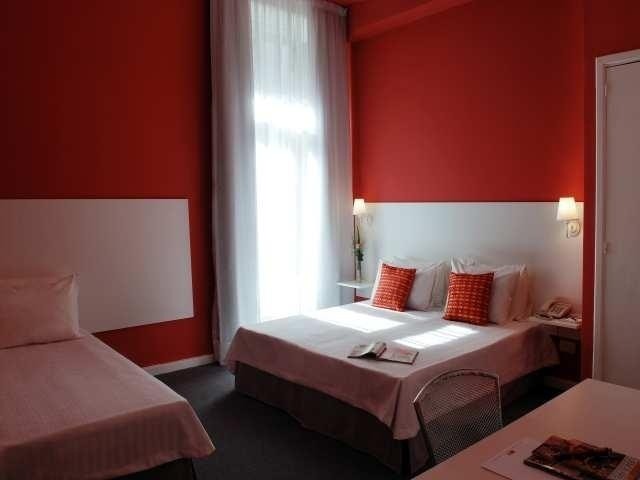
[
  {"left": 352, "top": 197, "right": 367, "bottom": 221},
  {"left": 557, "top": 198, "right": 582, "bottom": 237}
]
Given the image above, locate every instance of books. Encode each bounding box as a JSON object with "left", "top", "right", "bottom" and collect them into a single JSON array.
[
  {"left": 347, "top": 340, "right": 419, "bottom": 365},
  {"left": 523, "top": 434, "right": 640, "bottom": 479}
]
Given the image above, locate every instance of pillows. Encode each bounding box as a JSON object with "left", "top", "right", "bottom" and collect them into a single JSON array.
[
  {"left": 450, "top": 259, "right": 529, "bottom": 317},
  {"left": 442, "top": 270, "right": 494, "bottom": 326},
  {"left": 0, "top": 272, "right": 84, "bottom": 349},
  {"left": 369, "top": 262, "right": 436, "bottom": 312},
  {"left": 451, "top": 256, "right": 520, "bottom": 326},
  {"left": 432, "top": 261, "right": 451, "bottom": 310},
  {"left": 371, "top": 262, "right": 415, "bottom": 313}
]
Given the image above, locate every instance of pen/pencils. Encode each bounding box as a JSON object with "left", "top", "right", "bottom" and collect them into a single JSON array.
[{"left": 551, "top": 448, "right": 613, "bottom": 458}]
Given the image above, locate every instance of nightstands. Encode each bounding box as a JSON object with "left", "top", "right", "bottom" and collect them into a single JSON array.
[
  {"left": 336, "top": 280, "right": 372, "bottom": 302},
  {"left": 535, "top": 310, "right": 582, "bottom": 398}
]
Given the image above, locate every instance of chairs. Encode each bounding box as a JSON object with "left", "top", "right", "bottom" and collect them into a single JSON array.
[{"left": 413, "top": 368, "right": 504, "bottom": 464}]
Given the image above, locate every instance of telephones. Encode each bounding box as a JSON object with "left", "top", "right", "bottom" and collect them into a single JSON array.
[{"left": 536, "top": 299, "right": 573, "bottom": 318}]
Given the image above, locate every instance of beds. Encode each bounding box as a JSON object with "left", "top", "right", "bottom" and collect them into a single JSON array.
[
  {"left": 226, "top": 300, "right": 560, "bottom": 470},
  {"left": 0, "top": 329, "right": 216, "bottom": 480}
]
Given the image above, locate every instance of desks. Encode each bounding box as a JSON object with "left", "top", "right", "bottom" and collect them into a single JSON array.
[{"left": 414, "top": 377, "right": 640, "bottom": 480}]
